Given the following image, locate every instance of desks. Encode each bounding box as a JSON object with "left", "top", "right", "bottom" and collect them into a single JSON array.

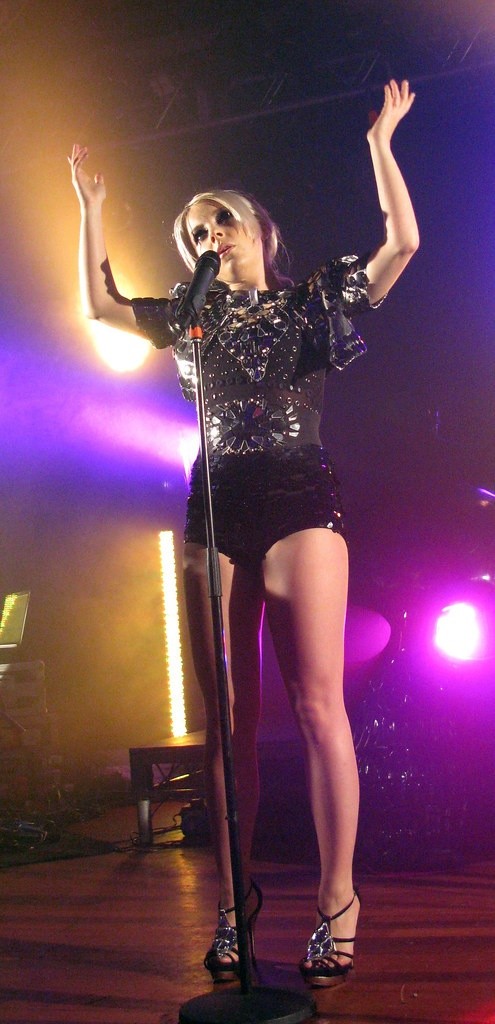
[{"left": 128, "top": 729, "right": 206, "bottom": 847}]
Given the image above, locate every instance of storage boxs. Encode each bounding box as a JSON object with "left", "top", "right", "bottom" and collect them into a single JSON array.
[{"left": 0, "top": 659, "right": 48, "bottom": 718}]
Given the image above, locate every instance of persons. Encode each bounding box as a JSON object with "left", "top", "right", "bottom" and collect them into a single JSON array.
[{"left": 67, "top": 79, "right": 420, "bottom": 987}]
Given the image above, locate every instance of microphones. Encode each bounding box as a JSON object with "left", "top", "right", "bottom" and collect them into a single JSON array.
[{"left": 175, "top": 251, "right": 221, "bottom": 331}]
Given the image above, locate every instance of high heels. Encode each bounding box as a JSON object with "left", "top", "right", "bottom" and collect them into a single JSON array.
[
  {"left": 203, "top": 875, "right": 264, "bottom": 983},
  {"left": 300, "top": 883, "right": 362, "bottom": 987}
]
